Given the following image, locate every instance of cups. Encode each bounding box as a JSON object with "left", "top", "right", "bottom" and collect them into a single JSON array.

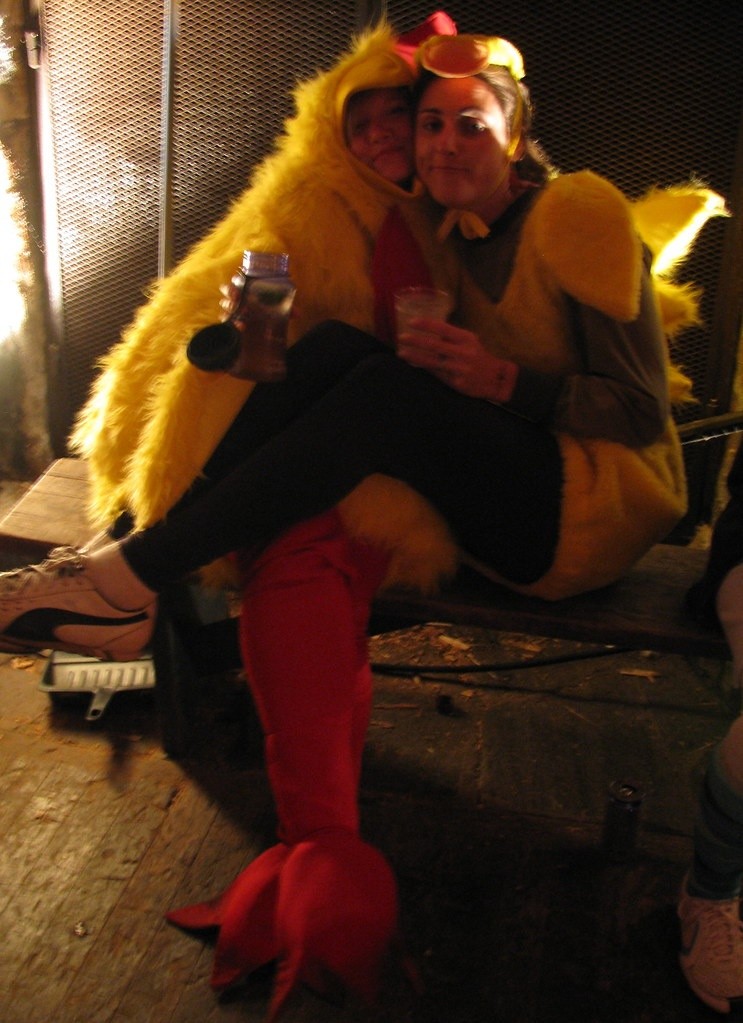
[
  {"left": 187, "top": 250, "right": 298, "bottom": 385},
  {"left": 392, "top": 287, "right": 453, "bottom": 368}
]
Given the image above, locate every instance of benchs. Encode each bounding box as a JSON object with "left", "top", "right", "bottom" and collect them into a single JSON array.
[{"left": 0, "top": 457, "right": 737, "bottom": 760}]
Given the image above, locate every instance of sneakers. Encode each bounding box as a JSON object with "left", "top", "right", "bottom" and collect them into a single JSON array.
[
  {"left": 0, "top": 542, "right": 161, "bottom": 664},
  {"left": 678, "top": 869, "right": 742, "bottom": 1015}
]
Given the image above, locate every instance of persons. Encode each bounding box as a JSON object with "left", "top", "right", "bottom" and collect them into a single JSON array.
[
  {"left": 676, "top": 432, "right": 743, "bottom": 1013},
  {"left": 1, "top": 35, "right": 689, "bottom": 1015}
]
